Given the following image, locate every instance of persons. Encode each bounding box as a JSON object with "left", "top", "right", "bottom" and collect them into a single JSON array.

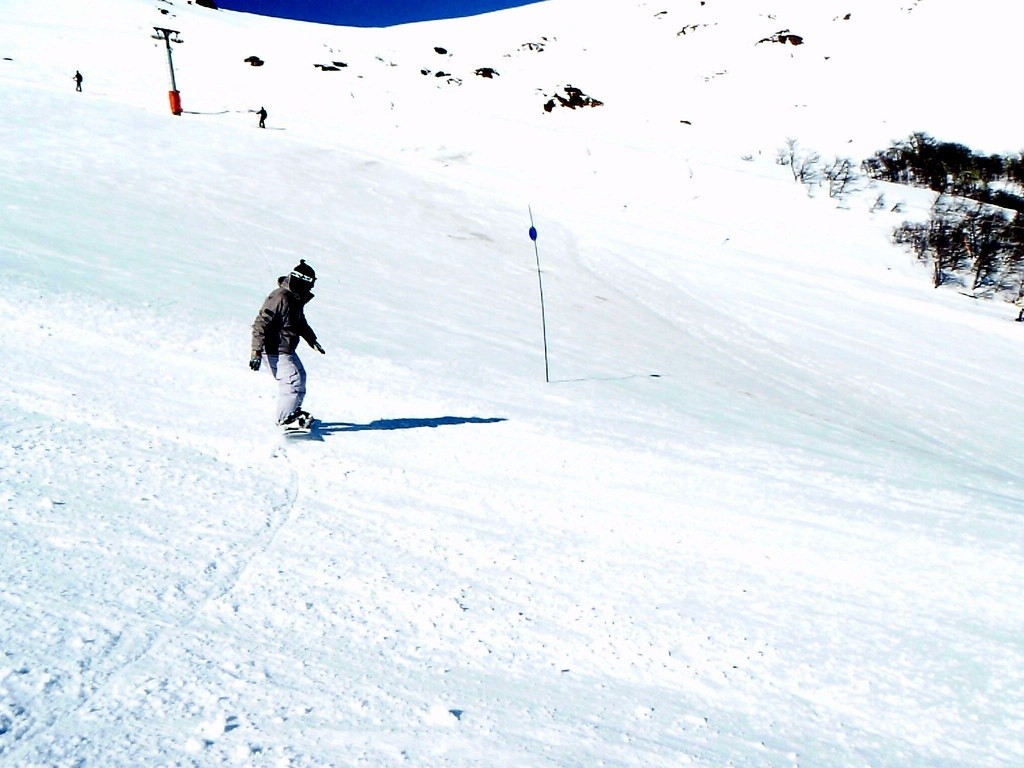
[
  {"left": 249, "top": 263, "right": 325, "bottom": 432},
  {"left": 73, "top": 70, "right": 82, "bottom": 91},
  {"left": 256, "top": 107, "right": 267, "bottom": 128}
]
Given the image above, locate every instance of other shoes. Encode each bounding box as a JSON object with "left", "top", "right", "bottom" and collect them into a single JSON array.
[{"left": 279, "top": 407, "right": 314, "bottom": 429}]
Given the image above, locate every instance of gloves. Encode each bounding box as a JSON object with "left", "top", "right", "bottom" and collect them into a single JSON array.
[
  {"left": 312, "top": 341, "right": 325, "bottom": 354},
  {"left": 249, "top": 351, "right": 262, "bottom": 371}
]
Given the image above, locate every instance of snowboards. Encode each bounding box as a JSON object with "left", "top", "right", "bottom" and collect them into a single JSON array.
[{"left": 285, "top": 426, "right": 314, "bottom": 436}]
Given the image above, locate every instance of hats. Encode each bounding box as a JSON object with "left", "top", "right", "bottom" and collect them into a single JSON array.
[{"left": 294, "top": 259, "right": 317, "bottom": 281}]
